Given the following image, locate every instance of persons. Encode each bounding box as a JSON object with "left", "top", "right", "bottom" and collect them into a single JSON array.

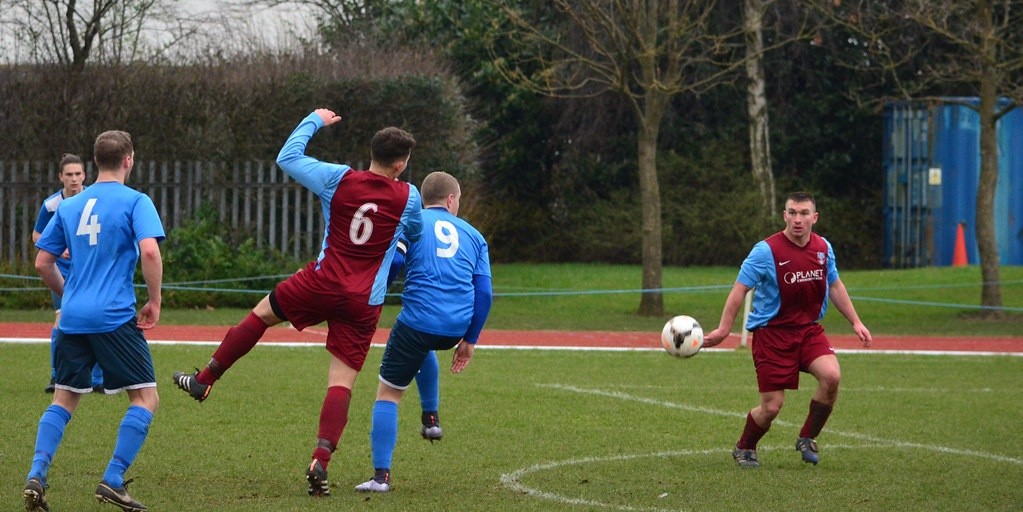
[
  {"left": 701, "top": 189, "right": 873, "bottom": 469},
  {"left": 356, "top": 171, "right": 491, "bottom": 488},
  {"left": 23, "top": 129, "right": 165, "bottom": 512},
  {"left": 172, "top": 108, "right": 423, "bottom": 496},
  {"left": 33, "top": 153, "right": 104, "bottom": 395}
]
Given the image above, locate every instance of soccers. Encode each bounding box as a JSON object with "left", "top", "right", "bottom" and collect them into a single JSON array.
[{"left": 661, "top": 315, "right": 704, "bottom": 359}]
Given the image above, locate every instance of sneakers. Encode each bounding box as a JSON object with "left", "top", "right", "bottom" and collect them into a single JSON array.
[
  {"left": 45, "top": 380, "right": 54, "bottom": 393},
  {"left": 306, "top": 459, "right": 329, "bottom": 496},
  {"left": 23, "top": 479, "right": 48, "bottom": 512},
  {"left": 94, "top": 478, "right": 148, "bottom": 512},
  {"left": 795, "top": 437, "right": 819, "bottom": 464},
  {"left": 172, "top": 367, "right": 212, "bottom": 403},
  {"left": 355, "top": 479, "right": 389, "bottom": 492},
  {"left": 421, "top": 423, "right": 442, "bottom": 440},
  {"left": 732, "top": 446, "right": 759, "bottom": 467}
]
